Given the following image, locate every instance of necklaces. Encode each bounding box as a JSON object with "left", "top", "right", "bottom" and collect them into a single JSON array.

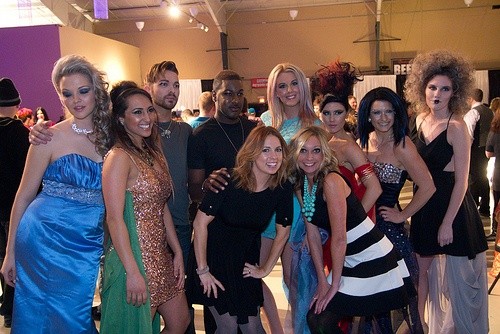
[
  {"left": 151, "top": 119, "right": 174, "bottom": 135},
  {"left": 298, "top": 169, "right": 321, "bottom": 223},
  {"left": 70, "top": 115, "right": 96, "bottom": 136}
]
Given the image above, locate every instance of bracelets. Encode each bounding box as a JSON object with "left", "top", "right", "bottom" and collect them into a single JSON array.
[
  {"left": 195, "top": 265, "right": 210, "bottom": 275},
  {"left": 358, "top": 163, "right": 376, "bottom": 184}
]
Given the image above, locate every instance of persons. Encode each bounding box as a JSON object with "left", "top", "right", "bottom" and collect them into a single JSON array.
[
  {"left": 255, "top": 61, "right": 327, "bottom": 334},
  {"left": 311, "top": 48, "right": 500, "bottom": 333},
  {"left": 1, "top": 55, "right": 112, "bottom": 334},
  {"left": 0, "top": 78, "right": 43, "bottom": 328},
  {"left": 189, "top": 126, "right": 295, "bottom": 334},
  {"left": 32, "top": 107, "right": 55, "bottom": 127},
  {"left": 285, "top": 127, "right": 417, "bottom": 334},
  {"left": 188, "top": 70, "right": 268, "bottom": 334},
  {"left": 181, "top": 109, "right": 196, "bottom": 124},
  {"left": 29, "top": 59, "right": 268, "bottom": 334},
  {"left": 102, "top": 80, "right": 191, "bottom": 333},
  {"left": 190, "top": 91, "right": 216, "bottom": 128},
  {"left": 17, "top": 108, "right": 34, "bottom": 130},
  {"left": 213, "top": 112, "right": 248, "bottom": 165},
  {"left": 171, "top": 110, "right": 183, "bottom": 123}
]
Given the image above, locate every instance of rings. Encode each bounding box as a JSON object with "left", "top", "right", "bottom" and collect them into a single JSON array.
[{"left": 248, "top": 270, "right": 251, "bottom": 273}]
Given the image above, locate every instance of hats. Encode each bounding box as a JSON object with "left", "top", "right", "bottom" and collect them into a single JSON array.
[
  {"left": 17, "top": 107, "right": 33, "bottom": 118},
  {"left": 248, "top": 109, "right": 256, "bottom": 115},
  {"left": 0, "top": 79, "right": 23, "bottom": 109}
]
